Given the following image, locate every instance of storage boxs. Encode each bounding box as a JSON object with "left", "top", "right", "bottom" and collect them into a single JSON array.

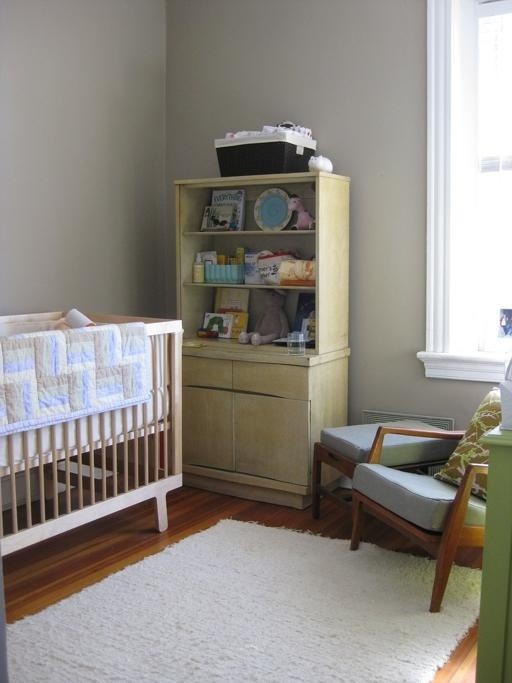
[{"left": 214, "top": 132, "right": 316, "bottom": 176}]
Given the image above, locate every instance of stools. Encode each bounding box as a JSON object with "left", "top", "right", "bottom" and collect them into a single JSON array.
[{"left": 313, "top": 418, "right": 460, "bottom": 540}]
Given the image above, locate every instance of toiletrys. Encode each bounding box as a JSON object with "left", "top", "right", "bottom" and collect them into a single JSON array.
[{"left": 208, "top": 248, "right": 244, "bottom": 264}]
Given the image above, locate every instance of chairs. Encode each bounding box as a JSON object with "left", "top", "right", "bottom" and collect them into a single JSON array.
[{"left": 350, "top": 425, "right": 489, "bottom": 613}]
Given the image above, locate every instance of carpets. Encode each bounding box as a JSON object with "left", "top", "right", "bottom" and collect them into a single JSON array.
[{"left": 6, "top": 519, "right": 482, "bottom": 683}]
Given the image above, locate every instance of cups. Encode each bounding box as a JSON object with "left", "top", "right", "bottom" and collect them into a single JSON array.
[{"left": 287, "top": 332, "right": 306, "bottom": 356}]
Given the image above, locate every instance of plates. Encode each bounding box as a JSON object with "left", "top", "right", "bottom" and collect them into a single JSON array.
[{"left": 253, "top": 188, "right": 292, "bottom": 231}]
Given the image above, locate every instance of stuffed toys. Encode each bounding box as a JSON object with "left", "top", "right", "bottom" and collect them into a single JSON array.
[
  {"left": 237, "top": 287, "right": 289, "bottom": 346},
  {"left": 287, "top": 194, "right": 316, "bottom": 230}
]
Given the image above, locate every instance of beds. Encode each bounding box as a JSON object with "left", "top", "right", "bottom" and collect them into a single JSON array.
[{"left": 0, "top": 310, "right": 182, "bottom": 555}]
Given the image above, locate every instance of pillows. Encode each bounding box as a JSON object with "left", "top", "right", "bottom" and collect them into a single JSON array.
[{"left": 434, "top": 387, "right": 502, "bottom": 501}]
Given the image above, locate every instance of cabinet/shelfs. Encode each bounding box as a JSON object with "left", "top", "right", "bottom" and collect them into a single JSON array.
[
  {"left": 172, "top": 171, "right": 349, "bottom": 354},
  {"left": 172, "top": 354, "right": 350, "bottom": 512}
]
